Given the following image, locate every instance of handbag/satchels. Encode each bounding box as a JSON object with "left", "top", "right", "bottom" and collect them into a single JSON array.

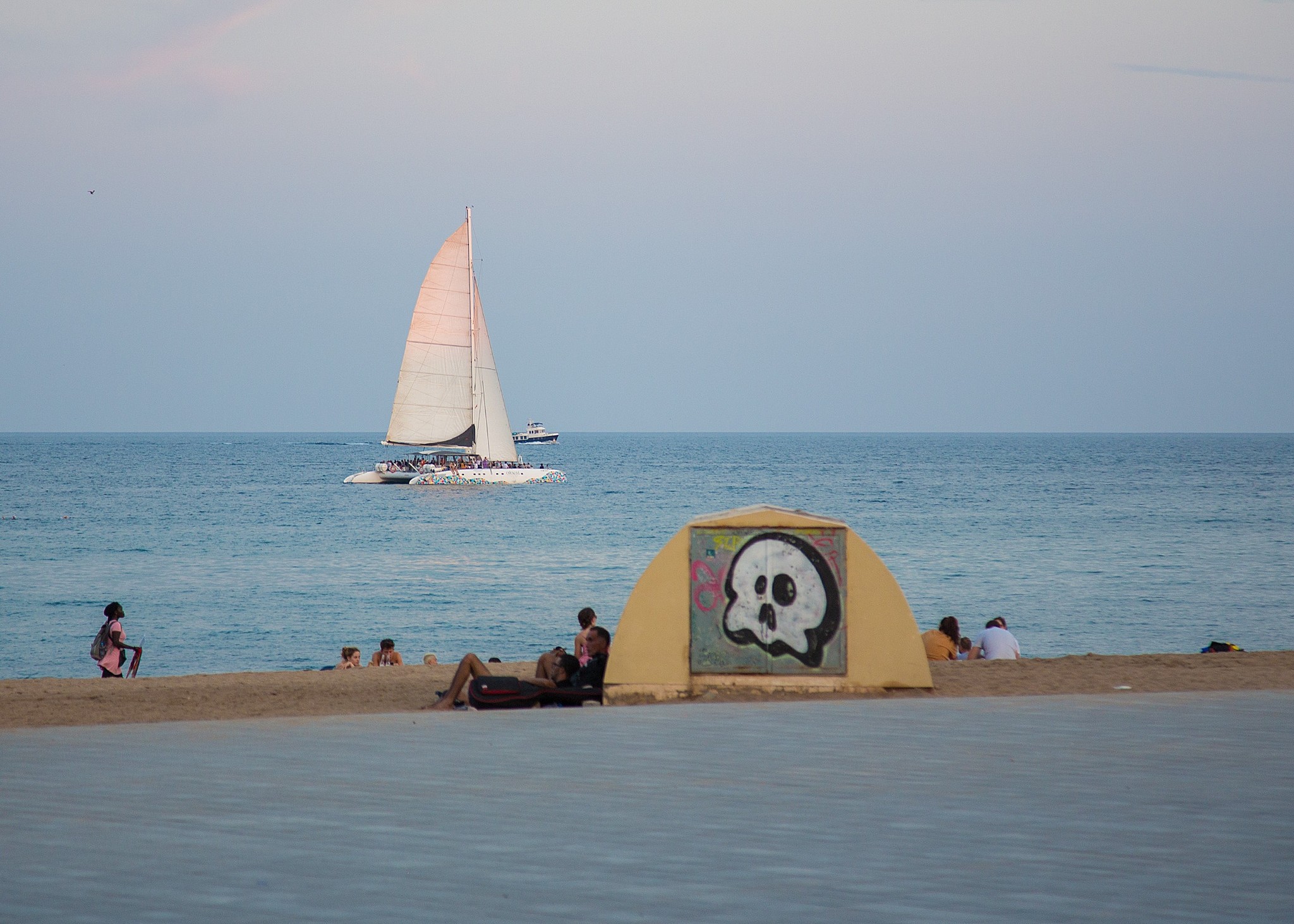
[{"left": 118, "top": 648, "right": 127, "bottom": 667}]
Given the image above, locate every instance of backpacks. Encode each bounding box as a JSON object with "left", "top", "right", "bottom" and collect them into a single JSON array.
[{"left": 90, "top": 621, "right": 122, "bottom": 661}]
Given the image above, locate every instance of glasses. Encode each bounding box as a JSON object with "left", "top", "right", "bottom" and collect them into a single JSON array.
[
  {"left": 551, "top": 662, "right": 562, "bottom": 668},
  {"left": 586, "top": 637, "right": 591, "bottom": 641}
]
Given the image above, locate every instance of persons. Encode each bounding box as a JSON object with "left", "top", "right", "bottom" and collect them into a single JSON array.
[
  {"left": 535, "top": 627, "right": 613, "bottom": 688},
  {"left": 994, "top": 616, "right": 1010, "bottom": 631},
  {"left": 949, "top": 637, "right": 971, "bottom": 660},
  {"left": 574, "top": 606, "right": 610, "bottom": 668},
  {"left": 968, "top": 620, "right": 1021, "bottom": 660},
  {"left": 368, "top": 639, "right": 403, "bottom": 667},
  {"left": 97, "top": 602, "right": 139, "bottom": 678},
  {"left": 334, "top": 647, "right": 360, "bottom": 670},
  {"left": 379, "top": 456, "right": 533, "bottom": 470},
  {"left": 921, "top": 616, "right": 960, "bottom": 661},
  {"left": 423, "top": 653, "right": 580, "bottom": 710},
  {"left": 540, "top": 464, "right": 545, "bottom": 469},
  {"left": 424, "top": 654, "right": 438, "bottom": 664}
]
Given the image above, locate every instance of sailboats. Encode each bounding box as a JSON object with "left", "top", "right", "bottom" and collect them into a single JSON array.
[{"left": 343, "top": 207, "right": 570, "bottom": 487}]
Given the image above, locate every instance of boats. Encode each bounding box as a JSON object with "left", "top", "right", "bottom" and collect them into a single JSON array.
[{"left": 510, "top": 418, "right": 559, "bottom": 444}]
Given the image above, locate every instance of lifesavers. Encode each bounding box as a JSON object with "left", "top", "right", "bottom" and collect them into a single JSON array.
[{"left": 390, "top": 466, "right": 396, "bottom": 473}]
